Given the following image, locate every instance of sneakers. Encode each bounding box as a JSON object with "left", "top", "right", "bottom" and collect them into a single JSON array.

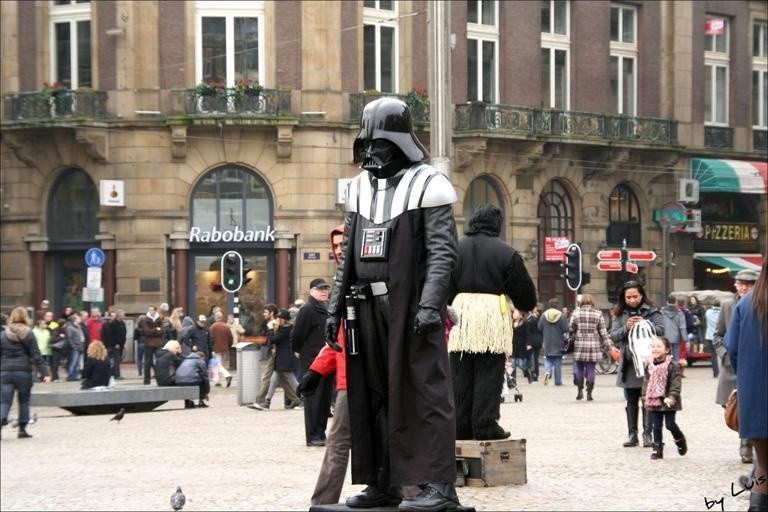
[
  {"left": 543, "top": 372, "right": 549, "bottom": 385},
  {"left": 739, "top": 476, "right": 751, "bottom": 490},
  {"left": 247, "top": 401, "right": 270, "bottom": 410},
  {"left": 285, "top": 402, "right": 305, "bottom": 409},
  {"left": 215, "top": 376, "right": 232, "bottom": 387},
  {"left": 742, "top": 456, "right": 753, "bottom": 463},
  {"left": 189, "top": 400, "right": 209, "bottom": 407},
  {"left": 115, "top": 376, "right": 124, "bottom": 380}
]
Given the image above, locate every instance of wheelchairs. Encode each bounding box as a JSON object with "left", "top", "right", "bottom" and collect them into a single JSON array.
[{"left": 593, "top": 347, "right": 618, "bottom": 376}]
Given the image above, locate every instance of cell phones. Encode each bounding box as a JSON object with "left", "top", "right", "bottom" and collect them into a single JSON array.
[{"left": 630, "top": 312, "right": 636, "bottom": 317}]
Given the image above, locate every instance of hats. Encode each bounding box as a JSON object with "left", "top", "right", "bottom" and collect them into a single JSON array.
[
  {"left": 734, "top": 269, "right": 758, "bottom": 281},
  {"left": 275, "top": 308, "right": 291, "bottom": 321},
  {"left": 196, "top": 314, "right": 207, "bottom": 321},
  {"left": 310, "top": 278, "right": 331, "bottom": 289}
]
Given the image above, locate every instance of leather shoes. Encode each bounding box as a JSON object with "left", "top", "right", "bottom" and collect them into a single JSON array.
[{"left": 307, "top": 438, "right": 326, "bottom": 446}]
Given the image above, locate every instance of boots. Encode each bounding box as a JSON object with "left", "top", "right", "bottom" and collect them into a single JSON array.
[
  {"left": 693, "top": 343, "right": 704, "bottom": 353},
  {"left": 651, "top": 442, "right": 664, "bottom": 459},
  {"left": 576, "top": 380, "right": 583, "bottom": 399},
  {"left": 642, "top": 407, "right": 653, "bottom": 447},
  {"left": 525, "top": 368, "right": 538, "bottom": 383},
  {"left": 586, "top": 380, "right": 593, "bottom": 400},
  {"left": 623, "top": 406, "right": 639, "bottom": 446},
  {"left": 748, "top": 492, "right": 768, "bottom": 512},
  {"left": 674, "top": 433, "right": 687, "bottom": 455},
  {"left": 18, "top": 423, "right": 31, "bottom": 437}
]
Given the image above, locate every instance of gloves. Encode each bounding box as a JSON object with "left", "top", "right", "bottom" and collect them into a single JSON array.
[{"left": 296, "top": 370, "right": 321, "bottom": 398}]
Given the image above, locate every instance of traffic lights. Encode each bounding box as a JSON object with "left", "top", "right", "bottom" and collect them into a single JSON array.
[
  {"left": 220, "top": 248, "right": 245, "bottom": 296},
  {"left": 561, "top": 246, "right": 583, "bottom": 290}
]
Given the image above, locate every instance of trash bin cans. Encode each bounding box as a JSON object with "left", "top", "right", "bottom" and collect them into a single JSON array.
[{"left": 236, "top": 341, "right": 261, "bottom": 404}]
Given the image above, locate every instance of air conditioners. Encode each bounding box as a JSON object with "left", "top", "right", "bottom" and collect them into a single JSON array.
[
  {"left": 675, "top": 178, "right": 700, "bottom": 205},
  {"left": 684, "top": 209, "right": 703, "bottom": 233}
]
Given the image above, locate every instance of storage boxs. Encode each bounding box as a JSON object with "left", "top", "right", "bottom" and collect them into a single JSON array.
[{"left": 454, "top": 437, "right": 528, "bottom": 488}]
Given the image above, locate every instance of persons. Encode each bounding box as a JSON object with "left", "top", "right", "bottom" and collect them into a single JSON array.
[
  {"left": 294, "top": 223, "right": 348, "bottom": 511},
  {"left": 325, "top": 98, "right": 461, "bottom": 512},
  {"left": 1, "top": 277, "right": 337, "bottom": 447},
  {"left": 509, "top": 254, "right": 767, "bottom": 512},
  {"left": 444, "top": 203, "right": 537, "bottom": 439}
]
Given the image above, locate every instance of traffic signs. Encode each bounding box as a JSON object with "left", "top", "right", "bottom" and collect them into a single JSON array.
[
  {"left": 625, "top": 262, "right": 638, "bottom": 274},
  {"left": 627, "top": 252, "right": 656, "bottom": 261},
  {"left": 598, "top": 262, "right": 622, "bottom": 271},
  {"left": 597, "top": 251, "right": 621, "bottom": 259}
]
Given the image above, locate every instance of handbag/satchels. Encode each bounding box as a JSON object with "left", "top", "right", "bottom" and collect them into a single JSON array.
[
  {"left": 48, "top": 340, "right": 66, "bottom": 349},
  {"left": 724, "top": 388, "right": 738, "bottom": 431}
]
